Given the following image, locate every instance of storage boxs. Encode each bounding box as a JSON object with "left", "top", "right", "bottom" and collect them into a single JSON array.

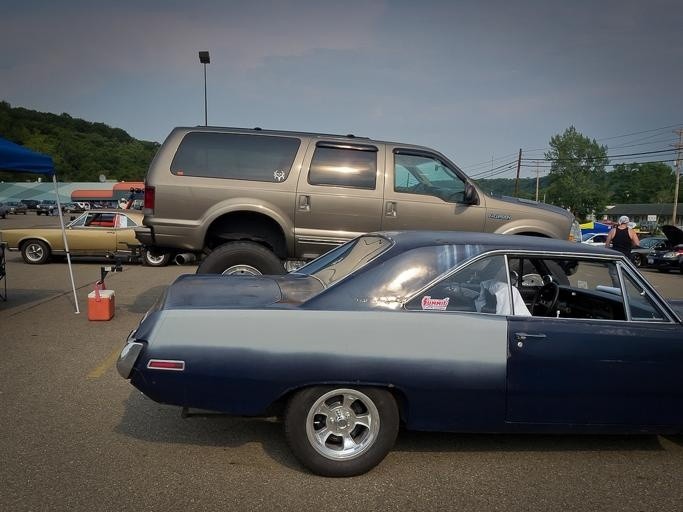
[{"left": 87, "top": 280, "right": 115, "bottom": 321}]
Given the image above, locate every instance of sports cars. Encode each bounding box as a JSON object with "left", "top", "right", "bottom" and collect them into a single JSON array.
[{"left": 117, "top": 229, "right": 682, "bottom": 479}]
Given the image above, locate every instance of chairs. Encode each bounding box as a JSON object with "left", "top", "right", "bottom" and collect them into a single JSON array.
[{"left": 443, "top": 280, "right": 534, "bottom": 316}]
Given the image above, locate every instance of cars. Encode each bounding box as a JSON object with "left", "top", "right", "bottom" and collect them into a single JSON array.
[
  {"left": 579, "top": 221, "right": 683, "bottom": 276},
  {"left": 0, "top": 188, "right": 168, "bottom": 266}
]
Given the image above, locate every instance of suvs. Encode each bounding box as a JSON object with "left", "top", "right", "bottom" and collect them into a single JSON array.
[{"left": 132, "top": 126, "right": 580, "bottom": 282}]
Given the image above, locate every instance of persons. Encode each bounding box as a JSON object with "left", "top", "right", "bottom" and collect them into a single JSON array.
[{"left": 604, "top": 215, "right": 640, "bottom": 290}]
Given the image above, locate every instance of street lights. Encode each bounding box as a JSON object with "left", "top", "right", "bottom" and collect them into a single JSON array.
[{"left": 197, "top": 50, "right": 211, "bottom": 127}]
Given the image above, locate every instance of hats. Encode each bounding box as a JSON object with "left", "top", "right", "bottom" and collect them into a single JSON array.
[{"left": 618, "top": 216, "right": 629, "bottom": 225}]
